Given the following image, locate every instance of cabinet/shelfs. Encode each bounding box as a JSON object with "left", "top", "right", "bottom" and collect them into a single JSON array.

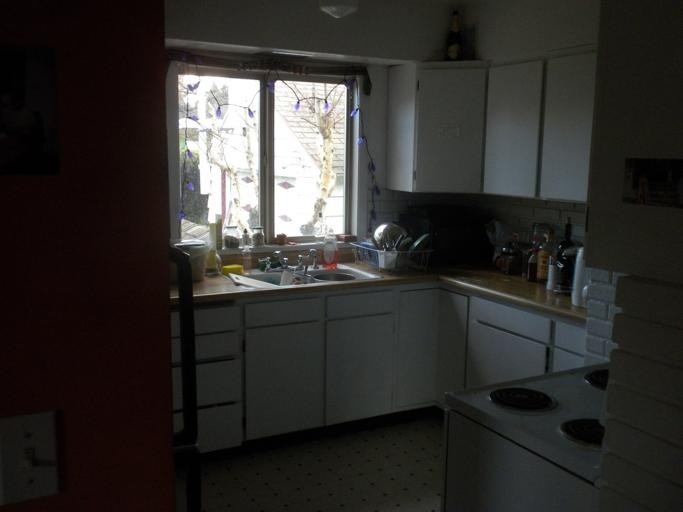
[
  {"left": 553, "top": 317, "right": 588, "bottom": 372},
  {"left": 467, "top": 294, "right": 553, "bottom": 388},
  {"left": 326, "top": 284, "right": 395, "bottom": 426},
  {"left": 395, "top": 287, "right": 466, "bottom": 412},
  {"left": 387, "top": 62, "right": 485, "bottom": 194},
  {"left": 484, "top": 53, "right": 601, "bottom": 204},
  {"left": 171, "top": 308, "right": 244, "bottom": 455},
  {"left": 244, "top": 289, "right": 326, "bottom": 443}
]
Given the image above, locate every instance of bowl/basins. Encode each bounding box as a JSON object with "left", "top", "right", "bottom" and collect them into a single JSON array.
[{"left": 372, "top": 221, "right": 407, "bottom": 250}]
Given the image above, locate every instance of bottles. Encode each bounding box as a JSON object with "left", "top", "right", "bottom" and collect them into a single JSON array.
[
  {"left": 445, "top": 11, "right": 462, "bottom": 61},
  {"left": 222, "top": 226, "right": 241, "bottom": 248},
  {"left": 538, "top": 223, "right": 553, "bottom": 282},
  {"left": 251, "top": 227, "right": 265, "bottom": 246},
  {"left": 527, "top": 249, "right": 538, "bottom": 281},
  {"left": 558, "top": 217, "right": 576, "bottom": 293}
]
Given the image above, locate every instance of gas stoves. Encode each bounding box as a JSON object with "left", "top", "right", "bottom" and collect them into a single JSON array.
[{"left": 444, "top": 360, "right": 610, "bottom": 469}]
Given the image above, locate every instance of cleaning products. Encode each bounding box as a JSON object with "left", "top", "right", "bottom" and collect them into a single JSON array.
[{"left": 320, "top": 238, "right": 339, "bottom": 269}]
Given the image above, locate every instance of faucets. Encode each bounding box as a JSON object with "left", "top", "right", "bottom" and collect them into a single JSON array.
[
  {"left": 272, "top": 250, "right": 288, "bottom": 269},
  {"left": 310, "top": 248, "right": 319, "bottom": 269}
]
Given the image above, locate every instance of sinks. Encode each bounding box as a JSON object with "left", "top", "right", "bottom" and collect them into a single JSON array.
[
  {"left": 244, "top": 270, "right": 313, "bottom": 287},
  {"left": 311, "top": 270, "right": 357, "bottom": 283}
]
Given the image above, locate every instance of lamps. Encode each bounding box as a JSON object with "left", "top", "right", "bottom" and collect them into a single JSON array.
[{"left": 316, "top": 0, "right": 359, "bottom": 19}]
